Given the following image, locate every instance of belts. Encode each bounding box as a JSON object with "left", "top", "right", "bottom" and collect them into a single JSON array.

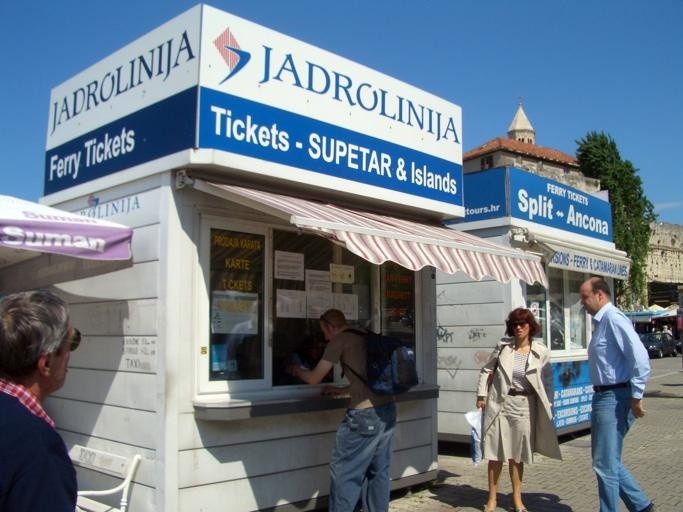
[
  {"left": 593, "top": 384, "right": 626, "bottom": 393},
  {"left": 509, "top": 389, "right": 529, "bottom": 396}
]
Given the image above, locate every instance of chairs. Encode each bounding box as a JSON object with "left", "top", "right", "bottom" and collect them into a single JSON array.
[{"left": 64, "top": 442, "right": 141, "bottom": 512}]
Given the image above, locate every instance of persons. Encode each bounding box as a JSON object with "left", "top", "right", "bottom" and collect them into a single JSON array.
[
  {"left": 292, "top": 309, "right": 397, "bottom": 511},
  {"left": 227, "top": 299, "right": 260, "bottom": 364},
  {"left": 580, "top": 277, "right": 656, "bottom": 512},
  {"left": 661, "top": 325, "right": 672, "bottom": 340},
  {"left": 0, "top": 292, "right": 77, "bottom": 512},
  {"left": 477, "top": 307, "right": 555, "bottom": 510}
]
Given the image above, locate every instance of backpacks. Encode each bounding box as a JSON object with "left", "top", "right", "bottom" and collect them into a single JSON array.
[{"left": 340, "top": 327, "right": 419, "bottom": 397}]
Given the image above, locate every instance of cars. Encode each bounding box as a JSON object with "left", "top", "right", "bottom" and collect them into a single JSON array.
[{"left": 640, "top": 333, "right": 680, "bottom": 358}]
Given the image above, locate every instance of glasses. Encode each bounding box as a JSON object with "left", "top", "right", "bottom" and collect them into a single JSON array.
[
  {"left": 510, "top": 320, "right": 527, "bottom": 329},
  {"left": 70, "top": 329, "right": 82, "bottom": 352}
]
